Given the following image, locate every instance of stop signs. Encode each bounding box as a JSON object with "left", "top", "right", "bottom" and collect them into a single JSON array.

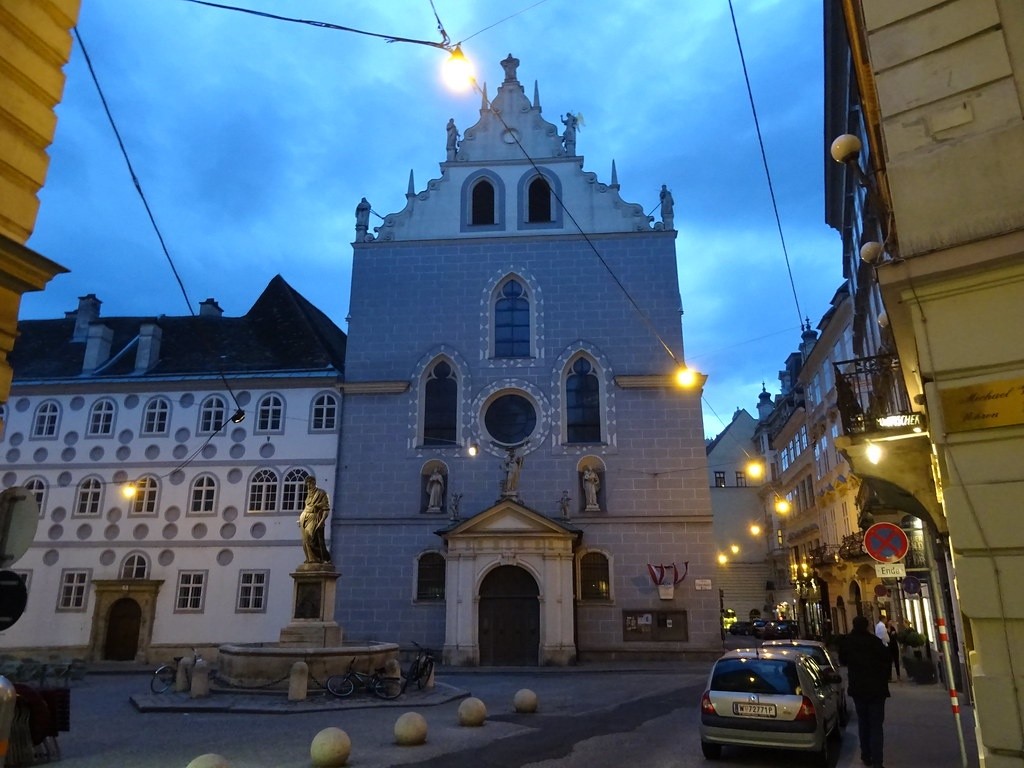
[{"left": 863, "top": 521, "right": 909, "bottom": 564}]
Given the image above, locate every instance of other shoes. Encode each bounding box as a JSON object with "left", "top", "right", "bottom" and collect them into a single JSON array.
[
  {"left": 897, "top": 678, "right": 902, "bottom": 681},
  {"left": 860, "top": 762, "right": 885, "bottom": 768}
]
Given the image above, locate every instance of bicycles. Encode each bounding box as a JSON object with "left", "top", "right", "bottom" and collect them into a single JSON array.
[
  {"left": 150, "top": 645, "right": 199, "bottom": 695},
  {"left": 325, "top": 656, "right": 406, "bottom": 702},
  {"left": 404, "top": 640, "right": 439, "bottom": 691}
]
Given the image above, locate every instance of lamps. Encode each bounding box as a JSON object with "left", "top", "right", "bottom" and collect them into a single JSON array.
[
  {"left": 860, "top": 240, "right": 885, "bottom": 283},
  {"left": 441, "top": 44, "right": 476, "bottom": 95},
  {"left": 122, "top": 480, "right": 138, "bottom": 500},
  {"left": 469, "top": 444, "right": 478, "bottom": 456},
  {"left": 830, "top": 134, "right": 879, "bottom": 204},
  {"left": 231, "top": 408, "right": 246, "bottom": 423}
]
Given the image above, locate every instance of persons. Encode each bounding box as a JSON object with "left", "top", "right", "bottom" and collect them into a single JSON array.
[
  {"left": 885, "top": 618, "right": 901, "bottom": 680},
  {"left": 499, "top": 447, "right": 524, "bottom": 492},
  {"left": 560, "top": 113, "right": 577, "bottom": 150},
  {"left": 355, "top": 197, "right": 371, "bottom": 229},
  {"left": 426, "top": 467, "right": 444, "bottom": 508},
  {"left": 449, "top": 489, "right": 463, "bottom": 522},
  {"left": 582, "top": 465, "right": 601, "bottom": 512},
  {"left": 446, "top": 118, "right": 460, "bottom": 152},
  {"left": 659, "top": 184, "right": 675, "bottom": 223},
  {"left": 297, "top": 475, "right": 332, "bottom": 564},
  {"left": 875, "top": 615, "right": 893, "bottom": 683},
  {"left": 837, "top": 615, "right": 898, "bottom": 768},
  {"left": 554, "top": 488, "right": 572, "bottom": 521}
]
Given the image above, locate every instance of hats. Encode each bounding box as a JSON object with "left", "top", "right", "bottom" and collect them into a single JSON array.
[
  {"left": 879, "top": 615, "right": 885, "bottom": 620},
  {"left": 853, "top": 615, "right": 869, "bottom": 626}
]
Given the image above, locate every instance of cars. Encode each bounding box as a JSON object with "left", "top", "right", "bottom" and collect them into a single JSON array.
[
  {"left": 727, "top": 617, "right": 800, "bottom": 641},
  {"left": 763, "top": 641, "right": 842, "bottom": 695},
  {"left": 698, "top": 648, "right": 845, "bottom": 768}
]
